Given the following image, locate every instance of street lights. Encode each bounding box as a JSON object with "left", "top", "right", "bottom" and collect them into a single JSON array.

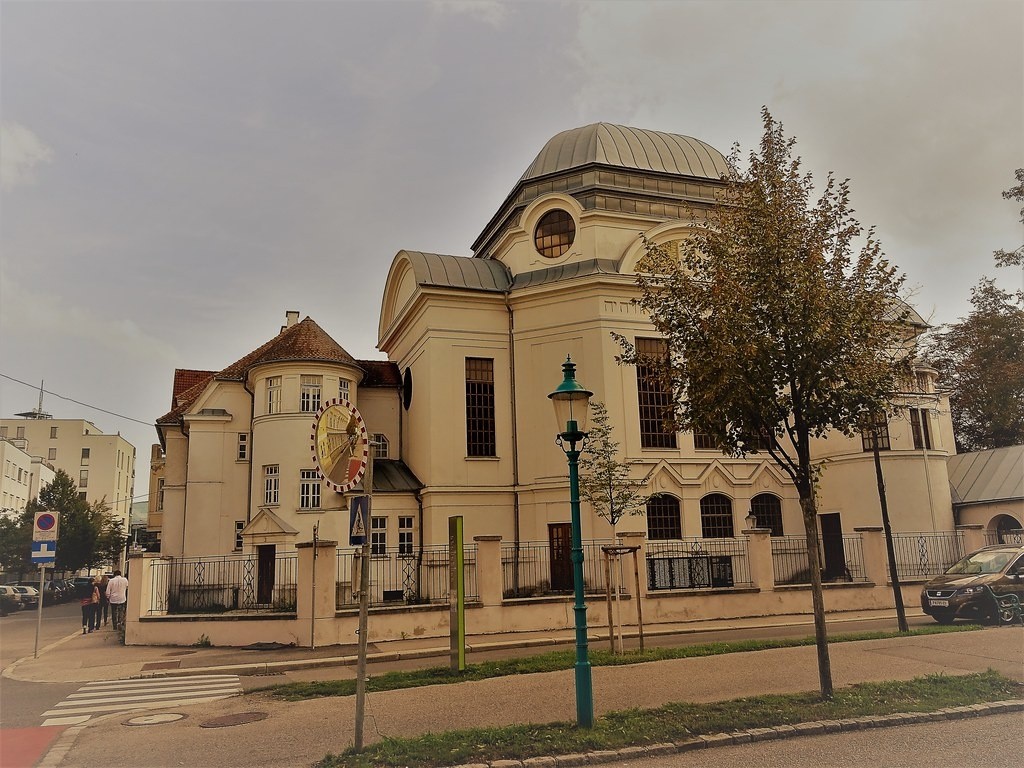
[{"left": 548, "top": 351, "right": 593, "bottom": 730}]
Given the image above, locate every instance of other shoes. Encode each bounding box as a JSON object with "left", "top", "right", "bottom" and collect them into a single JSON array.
[
  {"left": 82, "top": 628, "right": 87, "bottom": 634},
  {"left": 113, "top": 625, "right": 117, "bottom": 630},
  {"left": 88, "top": 630, "right": 93, "bottom": 633}
]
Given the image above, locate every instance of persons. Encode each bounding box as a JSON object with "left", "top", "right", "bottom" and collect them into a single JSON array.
[
  {"left": 81, "top": 575, "right": 110, "bottom": 634},
  {"left": 106, "top": 571, "right": 129, "bottom": 630}
]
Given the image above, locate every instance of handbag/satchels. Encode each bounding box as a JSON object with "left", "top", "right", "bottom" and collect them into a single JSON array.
[{"left": 92, "top": 586, "right": 97, "bottom": 603}]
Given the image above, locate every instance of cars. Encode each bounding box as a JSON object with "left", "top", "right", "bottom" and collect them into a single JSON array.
[{"left": 53, "top": 577, "right": 93, "bottom": 603}]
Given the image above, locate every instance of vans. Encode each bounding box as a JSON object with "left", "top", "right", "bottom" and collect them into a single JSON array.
[
  {"left": 5, "top": 581, "right": 54, "bottom": 594},
  {"left": 921, "top": 544, "right": 1024, "bottom": 625}
]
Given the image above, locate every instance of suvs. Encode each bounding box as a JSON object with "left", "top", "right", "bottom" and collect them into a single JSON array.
[
  {"left": 15, "top": 586, "right": 40, "bottom": 611},
  {"left": 0, "top": 587, "right": 21, "bottom": 603}
]
